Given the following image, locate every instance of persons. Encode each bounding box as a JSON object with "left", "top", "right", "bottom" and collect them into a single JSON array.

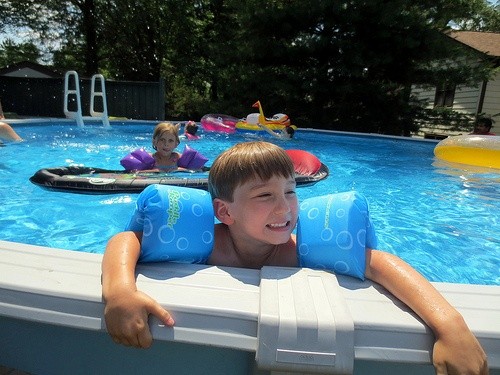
[
  {"left": 101, "top": 141, "right": 489, "bottom": 375},
  {"left": 257, "top": 122, "right": 295, "bottom": 138},
  {"left": 476, "top": 116, "right": 494, "bottom": 135},
  {"left": 131, "top": 122, "right": 195, "bottom": 172},
  {"left": 175, "top": 120, "right": 200, "bottom": 141}
]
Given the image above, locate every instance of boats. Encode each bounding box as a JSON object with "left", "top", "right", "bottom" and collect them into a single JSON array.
[{"left": 29, "top": 150, "right": 330, "bottom": 195}]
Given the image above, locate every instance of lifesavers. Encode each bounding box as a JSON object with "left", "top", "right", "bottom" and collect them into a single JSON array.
[
  {"left": 200, "top": 111, "right": 237, "bottom": 133},
  {"left": 236, "top": 99, "right": 298, "bottom": 133},
  {"left": 127, "top": 185, "right": 379, "bottom": 281},
  {"left": 433, "top": 133, "right": 500, "bottom": 169}
]
